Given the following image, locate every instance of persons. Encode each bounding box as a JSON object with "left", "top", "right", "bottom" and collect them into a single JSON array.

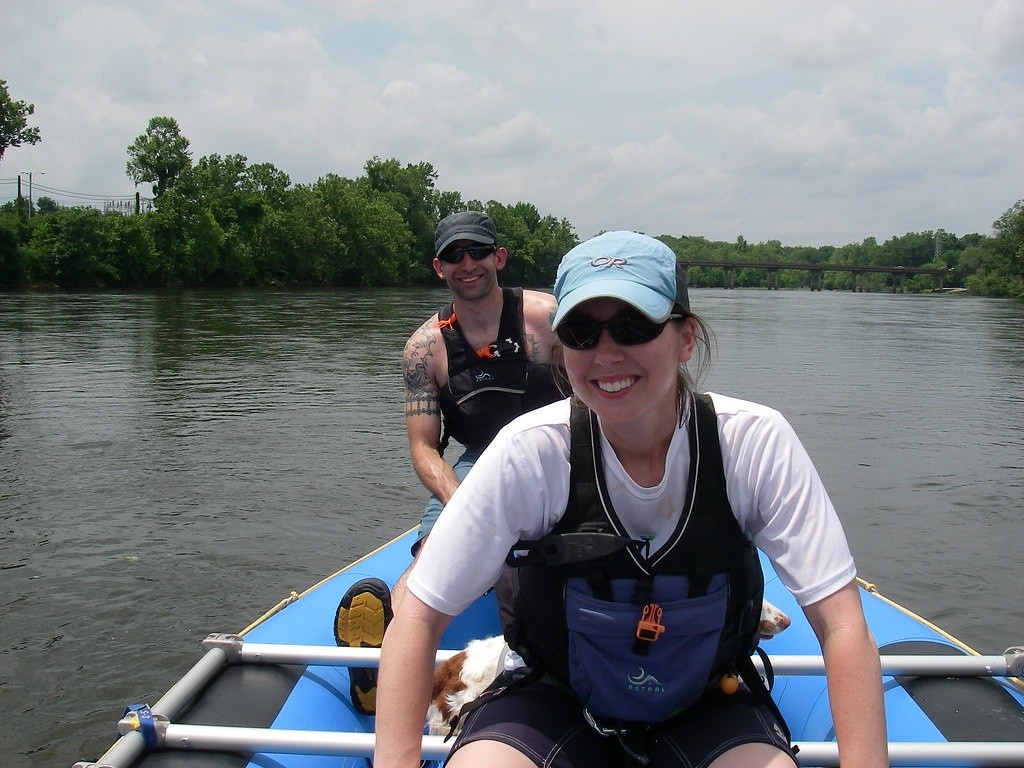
[
  {"left": 373, "top": 231, "right": 891, "bottom": 768},
  {"left": 332, "top": 211, "right": 564, "bottom": 716}
]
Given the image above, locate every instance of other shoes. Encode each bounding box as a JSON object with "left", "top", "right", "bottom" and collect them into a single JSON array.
[{"left": 332, "top": 578, "right": 393, "bottom": 714}]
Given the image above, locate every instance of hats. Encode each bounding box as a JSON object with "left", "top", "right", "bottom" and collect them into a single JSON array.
[
  {"left": 551, "top": 231, "right": 691, "bottom": 332},
  {"left": 435, "top": 211, "right": 496, "bottom": 258}
]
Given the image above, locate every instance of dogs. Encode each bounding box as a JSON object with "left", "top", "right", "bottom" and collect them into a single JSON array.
[{"left": 424, "top": 596, "right": 793, "bottom": 768}]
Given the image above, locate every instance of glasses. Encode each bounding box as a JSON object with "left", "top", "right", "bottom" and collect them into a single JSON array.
[
  {"left": 440, "top": 246, "right": 493, "bottom": 264},
  {"left": 556, "top": 308, "right": 682, "bottom": 350}
]
